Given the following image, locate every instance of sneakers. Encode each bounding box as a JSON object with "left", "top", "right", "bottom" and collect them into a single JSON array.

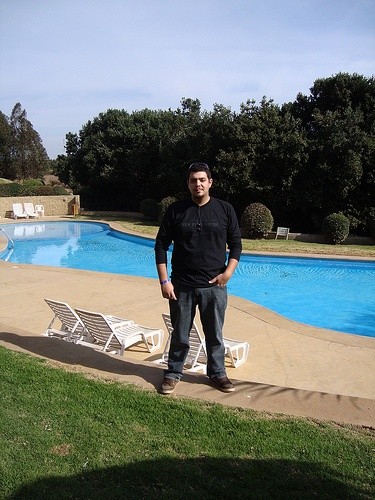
[
  {"left": 209, "top": 377, "right": 235, "bottom": 392},
  {"left": 160, "top": 379, "right": 180, "bottom": 394}
]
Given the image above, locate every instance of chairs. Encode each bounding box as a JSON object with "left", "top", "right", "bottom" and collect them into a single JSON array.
[
  {"left": 43, "top": 298, "right": 134, "bottom": 346},
  {"left": 275, "top": 226, "right": 290, "bottom": 240},
  {"left": 24, "top": 203, "right": 39, "bottom": 218},
  {"left": 35, "top": 205, "right": 44, "bottom": 216},
  {"left": 12, "top": 203, "right": 28, "bottom": 220},
  {"left": 74, "top": 308, "right": 164, "bottom": 356},
  {"left": 161, "top": 313, "right": 249, "bottom": 373}
]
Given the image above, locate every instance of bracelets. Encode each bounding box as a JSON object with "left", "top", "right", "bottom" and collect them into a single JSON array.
[{"left": 160, "top": 278, "right": 170, "bottom": 285}]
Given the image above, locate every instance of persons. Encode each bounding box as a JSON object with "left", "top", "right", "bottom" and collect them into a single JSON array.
[{"left": 152, "top": 160, "right": 244, "bottom": 395}]
